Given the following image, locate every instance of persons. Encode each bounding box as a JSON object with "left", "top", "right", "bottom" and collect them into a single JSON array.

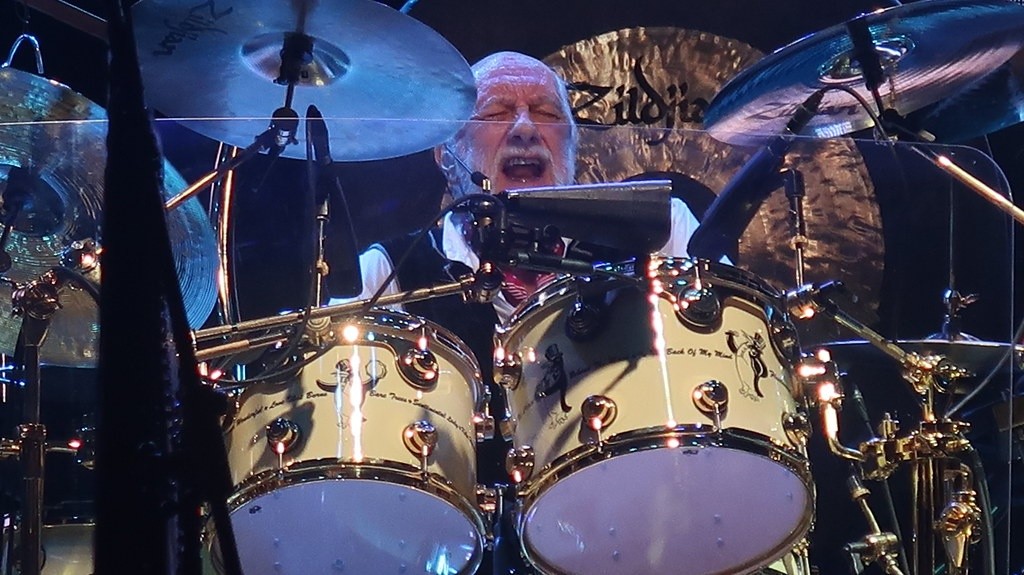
[{"left": 326, "top": 51, "right": 734, "bottom": 575}]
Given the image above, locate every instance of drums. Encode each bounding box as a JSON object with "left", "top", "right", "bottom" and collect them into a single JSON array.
[
  {"left": 1, "top": 515, "right": 101, "bottom": 575},
  {"left": 196, "top": 306, "right": 504, "bottom": 575},
  {"left": 491, "top": 255, "right": 820, "bottom": 575}
]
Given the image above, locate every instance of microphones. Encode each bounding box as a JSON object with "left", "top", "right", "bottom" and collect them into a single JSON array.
[
  {"left": 685, "top": 90, "right": 827, "bottom": 263},
  {"left": 444, "top": 146, "right": 489, "bottom": 187},
  {"left": 482, "top": 203, "right": 511, "bottom": 264},
  {"left": 307, "top": 106, "right": 363, "bottom": 299}
]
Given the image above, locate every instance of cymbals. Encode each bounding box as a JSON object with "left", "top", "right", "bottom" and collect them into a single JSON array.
[
  {"left": 0, "top": 63, "right": 220, "bottom": 371},
  {"left": 703, "top": 0, "right": 1022, "bottom": 146},
  {"left": 799, "top": 318, "right": 1024, "bottom": 386},
  {"left": 106, "top": 0, "right": 480, "bottom": 165}
]
